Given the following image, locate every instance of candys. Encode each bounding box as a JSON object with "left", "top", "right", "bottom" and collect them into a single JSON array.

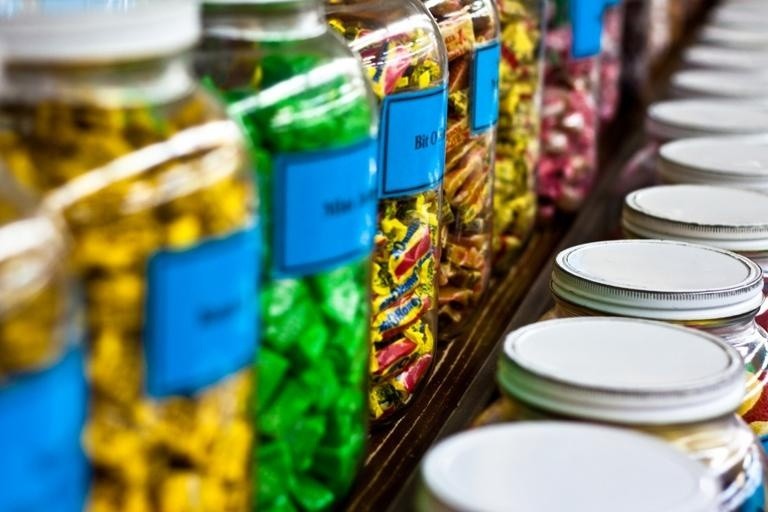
[{"left": 0, "top": 0, "right": 768, "bottom": 512}]
